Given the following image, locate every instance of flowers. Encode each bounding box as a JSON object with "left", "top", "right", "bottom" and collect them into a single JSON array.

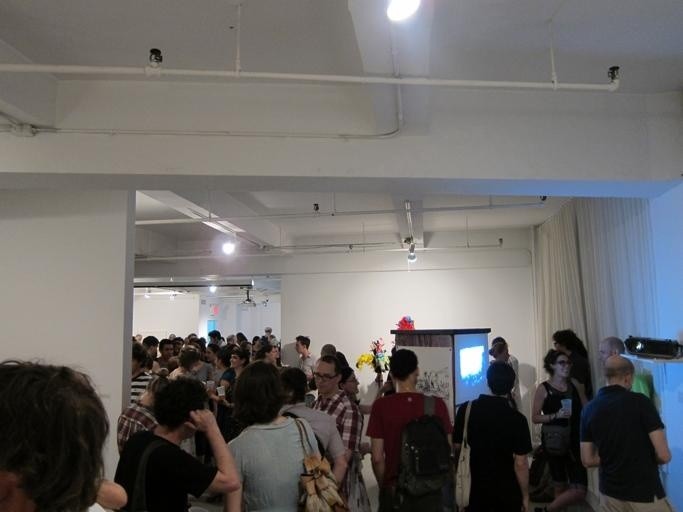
[
  {"left": 356, "top": 333, "right": 390, "bottom": 373},
  {"left": 396, "top": 315, "right": 415, "bottom": 330}
]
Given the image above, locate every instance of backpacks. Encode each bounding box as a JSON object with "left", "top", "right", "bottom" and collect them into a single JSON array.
[{"left": 395, "top": 396, "right": 452, "bottom": 495}]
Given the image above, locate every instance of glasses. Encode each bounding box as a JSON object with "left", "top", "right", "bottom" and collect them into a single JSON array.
[
  {"left": 344, "top": 378, "right": 357, "bottom": 383},
  {"left": 312, "top": 371, "right": 338, "bottom": 381},
  {"left": 551, "top": 360, "right": 573, "bottom": 367},
  {"left": 553, "top": 342, "right": 558, "bottom": 349}
]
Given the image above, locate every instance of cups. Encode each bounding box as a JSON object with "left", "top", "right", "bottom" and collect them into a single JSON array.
[{"left": 561, "top": 399, "right": 571, "bottom": 416}]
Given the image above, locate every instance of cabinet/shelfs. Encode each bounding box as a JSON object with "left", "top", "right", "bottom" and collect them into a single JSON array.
[{"left": 390, "top": 329, "right": 492, "bottom": 426}]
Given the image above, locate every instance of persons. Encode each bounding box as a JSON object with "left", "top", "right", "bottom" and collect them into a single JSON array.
[
  {"left": 365, "top": 349, "right": 454, "bottom": 511},
  {"left": 320, "top": 344, "right": 336, "bottom": 357},
  {"left": 552, "top": 328, "right": 593, "bottom": 401},
  {"left": 340, "top": 367, "right": 371, "bottom": 512},
  {"left": 224, "top": 360, "right": 323, "bottom": 512},
  {"left": 117, "top": 367, "right": 169, "bottom": 461},
  {"left": 132, "top": 327, "right": 280, "bottom": 360},
  {"left": 451, "top": 360, "right": 532, "bottom": 512},
  {"left": 489, "top": 337, "right": 522, "bottom": 413},
  {"left": 597, "top": 337, "right": 650, "bottom": 398},
  {"left": 168, "top": 344, "right": 199, "bottom": 457},
  {"left": 0, "top": 359, "right": 109, "bottom": 512},
  {"left": 208, "top": 351, "right": 233, "bottom": 433},
  {"left": 278, "top": 367, "right": 348, "bottom": 490},
  {"left": 310, "top": 354, "right": 365, "bottom": 490},
  {"left": 114, "top": 376, "right": 241, "bottom": 512},
  {"left": 129, "top": 340, "right": 152, "bottom": 408},
  {"left": 532, "top": 348, "right": 586, "bottom": 512},
  {"left": 94, "top": 472, "right": 128, "bottom": 512},
  {"left": 295, "top": 335, "right": 315, "bottom": 383},
  {"left": 580, "top": 355, "right": 674, "bottom": 511}
]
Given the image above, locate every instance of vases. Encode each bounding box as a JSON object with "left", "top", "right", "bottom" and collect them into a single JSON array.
[{"left": 375, "top": 373, "right": 384, "bottom": 398}]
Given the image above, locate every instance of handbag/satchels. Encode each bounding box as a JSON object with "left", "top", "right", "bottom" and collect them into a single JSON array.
[
  {"left": 293, "top": 418, "right": 349, "bottom": 512},
  {"left": 452, "top": 400, "right": 472, "bottom": 509}
]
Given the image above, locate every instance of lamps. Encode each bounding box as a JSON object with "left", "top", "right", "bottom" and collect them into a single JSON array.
[{"left": 407, "top": 245, "right": 419, "bottom": 262}]
